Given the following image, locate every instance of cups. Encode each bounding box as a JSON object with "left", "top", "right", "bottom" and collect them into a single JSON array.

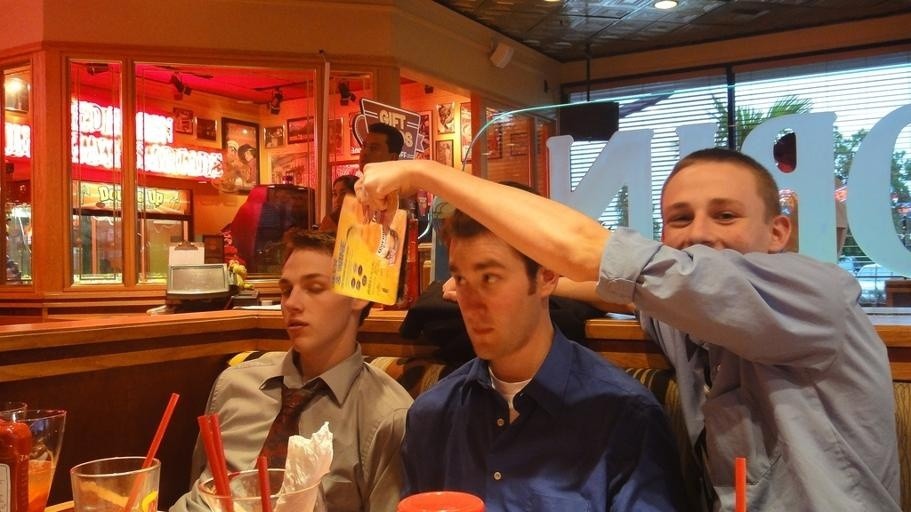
[
  {"left": 193, "top": 470, "right": 322, "bottom": 512},
  {"left": 70, "top": 454, "right": 163, "bottom": 511},
  {"left": 394, "top": 489, "right": 488, "bottom": 512},
  {"left": 0, "top": 401, "right": 69, "bottom": 511}
]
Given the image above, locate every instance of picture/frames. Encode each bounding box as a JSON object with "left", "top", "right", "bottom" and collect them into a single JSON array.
[
  {"left": 264, "top": 102, "right": 541, "bottom": 218},
  {"left": 173, "top": 108, "right": 261, "bottom": 195}
]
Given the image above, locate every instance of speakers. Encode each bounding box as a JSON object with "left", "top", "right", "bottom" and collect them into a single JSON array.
[{"left": 490, "top": 44, "right": 513, "bottom": 69}]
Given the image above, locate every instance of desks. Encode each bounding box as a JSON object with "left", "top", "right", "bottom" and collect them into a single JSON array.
[{"left": 44, "top": 500, "right": 167, "bottom": 512}]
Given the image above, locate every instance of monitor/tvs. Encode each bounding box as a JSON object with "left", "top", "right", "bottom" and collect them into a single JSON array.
[
  {"left": 166, "top": 264, "right": 230, "bottom": 299},
  {"left": 560, "top": 101, "right": 619, "bottom": 141}
]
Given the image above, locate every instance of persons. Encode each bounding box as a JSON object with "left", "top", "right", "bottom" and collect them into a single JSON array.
[
  {"left": 397, "top": 178, "right": 725, "bottom": 512},
  {"left": 356, "top": 126, "right": 403, "bottom": 168},
  {"left": 774, "top": 133, "right": 851, "bottom": 261},
  {"left": 158, "top": 224, "right": 412, "bottom": 512},
  {"left": 354, "top": 146, "right": 902, "bottom": 512},
  {"left": 321, "top": 172, "right": 357, "bottom": 232}
]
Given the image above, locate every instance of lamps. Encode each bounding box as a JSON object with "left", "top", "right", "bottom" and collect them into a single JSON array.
[
  {"left": 267, "top": 86, "right": 284, "bottom": 115},
  {"left": 339, "top": 78, "right": 356, "bottom": 106}
]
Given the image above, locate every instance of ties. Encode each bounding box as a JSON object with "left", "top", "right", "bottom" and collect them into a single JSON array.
[{"left": 247, "top": 378, "right": 327, "bottom": 512}]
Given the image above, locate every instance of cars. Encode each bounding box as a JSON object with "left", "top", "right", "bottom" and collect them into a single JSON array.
[
  {"left": 837, "top": 255, "right": 864, "bottom": 283},
  {"left": 849, "top": 263, "right": 911, "bottom": 308}
]
